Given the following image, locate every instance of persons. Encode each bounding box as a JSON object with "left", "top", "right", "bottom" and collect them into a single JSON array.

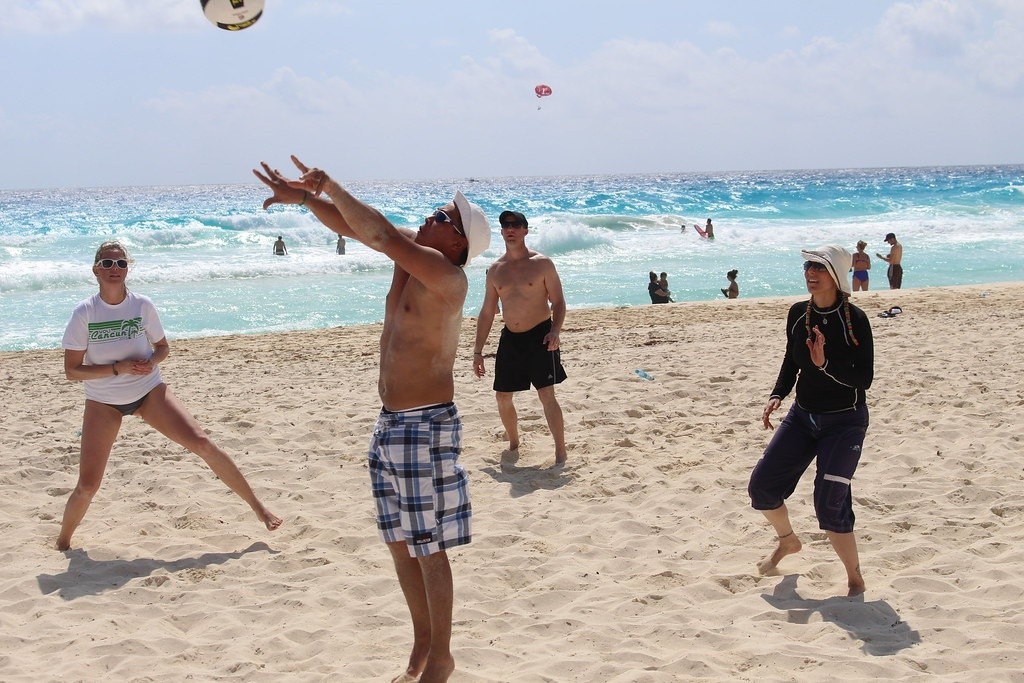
[
  {"left": 56, "top": 239, "right": 283, "bottom": 551},
  {"left": 680, "top": 225, "right": 687, "bottom": 234},
  {"left": 748, "top": 244, "right": 874, "bottom": 596},
  {"left": 272, "top": 235, "right": 288, "bottom": 255},
  {"left": 647, "top": 271, "right": 675, "bottom": 303},
  {"left": 876, "top": 233, "right": 903, "bottom": 289},
  {"left": 721, "top": 269, "right": 740, "bottom": 299},
  {"left": 473, "top": 210, "right": 571, "bottom": 467},
  {"left": 251, "top": 153, "right": 492, "bottom": 683},
  {"left": 336, "top": 234, "right": 346, "bottom": 254},
  {"left": 852, "top": 240, "right": 872, "bottom": 291},
  {"left": 703, "top": 218, "right": 714, "bottom": 240}
]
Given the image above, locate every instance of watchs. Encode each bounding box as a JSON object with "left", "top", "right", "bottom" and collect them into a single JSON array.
[{"left": 112, "top": 363, "right": 119, "bottom": 375}]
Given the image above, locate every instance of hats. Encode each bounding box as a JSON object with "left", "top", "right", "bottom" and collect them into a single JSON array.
[
  {"left": 454, "top": 189, "right": 491, "bottom": 268},
  {"left": 499, "top": 211, "right": 527, "bottom": 223},
  {"left": 802, "top": 243, "right": 852, "bottom": 297},
  {"left": 884, "top": 233, "right": 895, "bottom": 242}
]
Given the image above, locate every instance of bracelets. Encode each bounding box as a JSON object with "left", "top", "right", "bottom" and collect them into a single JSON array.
[
  {"left": 474, "top": 353, "right": 481, "bottom": 354},
  {"left": 298, "top": 191, "right": 308, "bottom": 206}
]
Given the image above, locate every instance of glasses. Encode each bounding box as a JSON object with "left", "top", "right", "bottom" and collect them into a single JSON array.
[
  {"left": 95, "top": 258, "right": 128, "bottom": 270},
  {"left": 803, "top": 260, "right": 828, "bottom": 272},
  {"left": 501, "top": 222, "right": 524, "bottom": 229},
  {"left": 433, "top": 208, "right": 462, "bottom": 235}
]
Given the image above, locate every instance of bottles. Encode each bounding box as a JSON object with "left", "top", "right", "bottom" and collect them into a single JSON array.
[
  {"left": 635, "top": 370, "right": 654, "bottom": 381},
  {"left": 882, "top": 313, "right": 888, "bottom": 316}
]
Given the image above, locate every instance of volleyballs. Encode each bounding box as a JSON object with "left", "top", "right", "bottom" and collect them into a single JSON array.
[{"left": 200, "top": 0, "right": 267, "bottom": 32}]
